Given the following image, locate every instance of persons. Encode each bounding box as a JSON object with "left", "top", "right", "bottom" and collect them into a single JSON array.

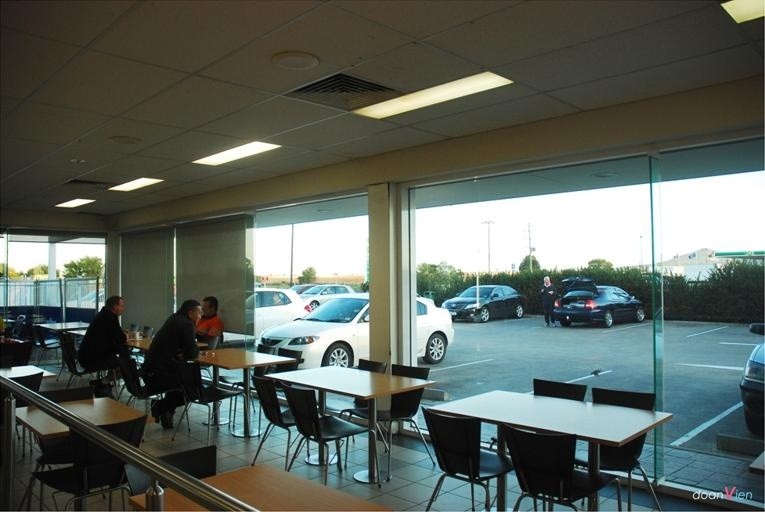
[
  {"left": 79, "top": 297, "right": 146, "bottom": 399},
  {"left": 272, "top": 293, "right": 283, "bottom": 304},
  {"left": 142, "top": 300, "right": 202, "bottom": 428},
  {"left": 541, "top": 276, "right": 557, "bottom": 327},
  {"left": 195, "top": 297, "right": 224, "bottom": 345}
]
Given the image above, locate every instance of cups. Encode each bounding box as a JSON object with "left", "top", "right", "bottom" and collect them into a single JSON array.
[{"left": 131, "top": 332, "right": 141, "bottom": 339}]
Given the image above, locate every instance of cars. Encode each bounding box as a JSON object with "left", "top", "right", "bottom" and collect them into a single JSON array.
[
  {"left": 738, "top": 319, "right": 765, "bottom": 439},
  {"left": 256, "top": 283, "right": 261, "bottom": 288},
  {"left": 299, "top": 284, "right": 357, "bottom": 310},
  {"left": 287, "top": 285, "right": 313, "bottom": 295},
  {"left": 552, "top": 276, "right": 646, "bottom": 329},
  {"left": 252, "top": 294, "right": 456, "bottom": 374},
  {"left": 65, "top": 289, "right": 106, "bottom": 312},
  {"left": 440, "top": 283, "right": 528, "bottom": 324},
  {"left": 224, "top": 288, "right": 315, "bottom": 346}
]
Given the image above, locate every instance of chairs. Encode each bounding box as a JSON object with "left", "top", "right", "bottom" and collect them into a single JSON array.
[{"left": 0, "top": 314, "right": 677, "bottom": 511}]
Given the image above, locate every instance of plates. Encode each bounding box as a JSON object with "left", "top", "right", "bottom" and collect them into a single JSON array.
[{"left": 129, "top": 338, "right": 143, "bottom": 341}]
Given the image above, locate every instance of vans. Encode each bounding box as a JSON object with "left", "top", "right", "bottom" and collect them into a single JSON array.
[{"left": 261, "top": 282, "right": 289, "bottom": 288}]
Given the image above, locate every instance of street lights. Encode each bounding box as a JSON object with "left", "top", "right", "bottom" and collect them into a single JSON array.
[{"left": 484, "top": 219, "right": 496, "bottom": 272}]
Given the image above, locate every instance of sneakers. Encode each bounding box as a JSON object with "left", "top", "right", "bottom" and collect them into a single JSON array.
[
  {"left": 160, "top": 409, "right": 177, "bottom": 430},
  {"left": 150, "top": 399, "right": 163, "bottom": 424}
]
